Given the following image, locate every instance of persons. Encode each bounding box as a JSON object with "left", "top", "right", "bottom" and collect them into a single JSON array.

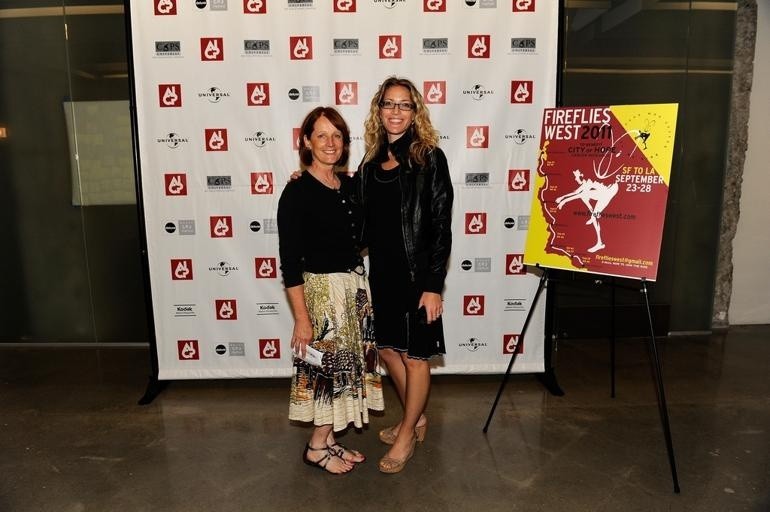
[
  {"left": 286, "top": 77, "right": 453, "bottom": 472},
  {"left": 276, "top": 108, "right": 386, "bottom": 476}
]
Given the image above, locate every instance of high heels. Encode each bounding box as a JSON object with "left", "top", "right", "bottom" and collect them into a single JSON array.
[
  {"left": 378, "top": 421, "right": 427, "bottom": 444},
  {"left": 379, "top": 437, "right": 417, "bottom": 476}
]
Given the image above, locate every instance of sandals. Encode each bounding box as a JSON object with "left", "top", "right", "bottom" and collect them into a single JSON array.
[
  {"left": 301, "top": 442, "right": 356, "bottom": 476},
  {"left": 328, "top": 443, "right": 367, "bottom": 465}
]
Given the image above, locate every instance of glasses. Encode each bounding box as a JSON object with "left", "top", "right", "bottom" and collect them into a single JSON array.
[{"left": 379, "top": 100, "right": 415, "bottom": 111}]
